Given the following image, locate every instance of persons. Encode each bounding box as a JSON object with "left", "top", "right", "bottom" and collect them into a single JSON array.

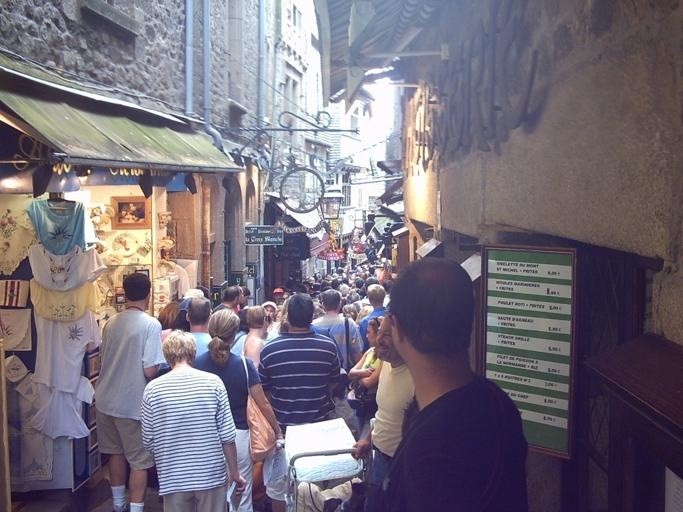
[
  {"left": 364, "top": 255, "right": 531, "bottom": 511},
  {"left": 128, "top": 202, "right": 144, "bottom": 218},
  {"left": 92, "top": 272, "right": 167, "bottom": 512}
]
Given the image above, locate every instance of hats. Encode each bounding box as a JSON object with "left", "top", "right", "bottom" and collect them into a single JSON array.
[
  {"left": 273, "top": 288, "right": 284, "bottom": 294},
  {"left": 262, "top": 300, "right": 278, "bottom": 315}
]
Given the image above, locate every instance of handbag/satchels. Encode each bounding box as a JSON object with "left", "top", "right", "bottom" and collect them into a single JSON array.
[
  {"left": 246, "top": 395, "right": 276, "bottom": 464},
  {"left": 347, "top": 389, "right": 361, "bottom": 410}
]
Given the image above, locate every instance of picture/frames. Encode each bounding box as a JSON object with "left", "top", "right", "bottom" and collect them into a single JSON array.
[{"left": 109, "top": 195, "right": 152, "bottom": 229}]
